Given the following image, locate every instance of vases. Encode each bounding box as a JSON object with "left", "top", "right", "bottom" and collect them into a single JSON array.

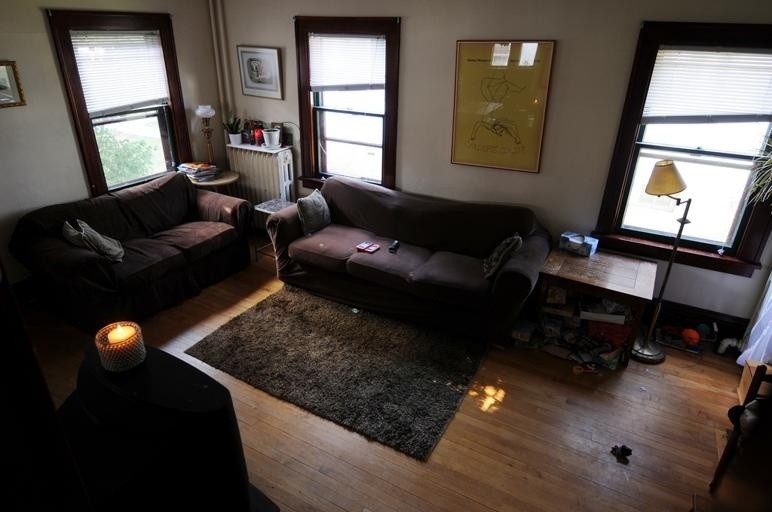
[{"left": 261, "top": 129, "right": 281, "bottom": 147}]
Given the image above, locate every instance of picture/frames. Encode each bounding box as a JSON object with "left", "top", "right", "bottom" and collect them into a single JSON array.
[
  {"left": 450, "top": 39, "right": 556, "bottom": 174},
  {"left": 0, "top": 59, "right": 26, "bottom": 109},
  {"left": 236, "top": 44, "right": 283, "bottom": 101}
]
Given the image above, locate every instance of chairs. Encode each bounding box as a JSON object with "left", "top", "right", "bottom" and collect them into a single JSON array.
[{"left": 708, "top": 364, "right": 772, "bottom": 491}]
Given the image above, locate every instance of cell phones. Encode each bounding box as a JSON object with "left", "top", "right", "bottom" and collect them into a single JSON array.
[
  {"left": 355, "top": 241, "right": 373, "bottom": 250},
  {"left": 365, "top": 244, "right": 380, "bottom": 252}
]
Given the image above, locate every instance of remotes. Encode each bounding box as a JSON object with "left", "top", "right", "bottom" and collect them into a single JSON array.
[{"left": 388, "top": 240, "right": 400, "bottom": 252}]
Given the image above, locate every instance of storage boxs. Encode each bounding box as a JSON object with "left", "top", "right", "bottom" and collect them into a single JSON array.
[
  {"left": 588, "top": 317, "right": 632, "bottom": 359},
  {"left": 736, "top": 359, "right": 772, "bottom": 405}
]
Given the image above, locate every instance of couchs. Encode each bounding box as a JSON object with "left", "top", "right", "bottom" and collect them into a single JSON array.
[
  {"left": 6, "top": 171, "right": 258, "bottom": 331},
  {"left": 265, "top": 172, "right": 551, "bottom": 351}
]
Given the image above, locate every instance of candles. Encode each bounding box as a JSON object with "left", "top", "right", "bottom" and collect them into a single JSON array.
[{"left": 108, "top": 326, "right": 134, "bottom": 344}]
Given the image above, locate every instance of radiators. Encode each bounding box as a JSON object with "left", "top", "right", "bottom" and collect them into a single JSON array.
[{"left": 225, "top": 145, "right": 297, "bottom": 232}]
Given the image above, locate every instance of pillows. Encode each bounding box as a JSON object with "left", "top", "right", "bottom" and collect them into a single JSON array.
[
  {"left": 63, "top": 217, "right": 125, "bottom": 263},
  {"left": 480, "top": 230, "right": 524, "bottom": 279},
  {"left": 294, "top": 187, "right": 333, "bottom": 236}
]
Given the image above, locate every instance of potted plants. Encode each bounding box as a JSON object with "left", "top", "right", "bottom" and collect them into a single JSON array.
[{"left": 220, "top": 116, "right": 245, "bottom": 145}]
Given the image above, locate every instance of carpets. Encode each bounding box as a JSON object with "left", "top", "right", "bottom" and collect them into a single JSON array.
[{"left": 185, "top": 284, "right": 492, "bottom": 464}]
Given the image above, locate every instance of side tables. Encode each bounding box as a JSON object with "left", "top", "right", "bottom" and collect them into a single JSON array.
[
  {"left": 187, "top": 171, "right": 241, "bottom": 193},
  {"left": 538, "top": 245, "right": 658, "bottom": 369}
]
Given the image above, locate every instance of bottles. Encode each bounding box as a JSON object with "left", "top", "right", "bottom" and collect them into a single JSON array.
[{"left": 250, "top": 129, "right": 255, "bottom": 146}]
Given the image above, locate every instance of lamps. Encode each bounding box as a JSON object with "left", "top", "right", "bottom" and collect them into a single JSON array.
[
  {"left": 194, "top": 104, "right": 219, "bottom": 167},
  {"left": 629, "top": 159, "right": 691, "bottom": 366}
]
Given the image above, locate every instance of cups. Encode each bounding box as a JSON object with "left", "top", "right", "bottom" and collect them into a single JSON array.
[{"left": 254, "top": 128, "right": 262, "bottom": 145}]
